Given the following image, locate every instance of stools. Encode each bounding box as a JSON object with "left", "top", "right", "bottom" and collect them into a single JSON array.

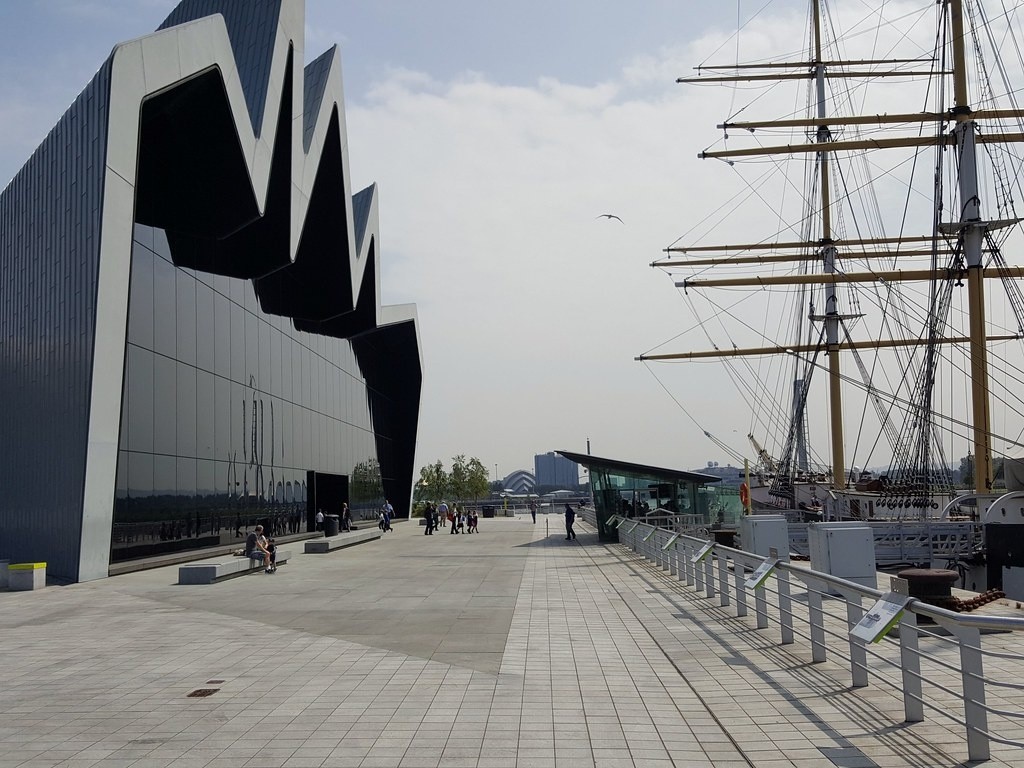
[{"left": 8, "top": 562, "right": 47, "bottom": 591}]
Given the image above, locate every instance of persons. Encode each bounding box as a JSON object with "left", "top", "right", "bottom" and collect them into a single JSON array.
[
  {"left": 467, "top": 510, "right": 475, "bottom": 534},
  {"left": 471, "top": 511, "right": 479, "bottom": 533},
  {"left": 450, "top": 502, "right": 459, "bottom": 534},
  {"left": 565, "top": 503, "right": 576, "bottom": 540},
  {"left": 530, "top": 500, "right": 539, "bottom": 524},
  {"left": 620, "top": 498, "right": 673, "bottom": 531},
  {"left": 245, "top": 525, "right": 277, "bottom": 574},
  {"left": 424, "top": 502, "right": 439, "bottom": 535},
  {"left": 438, "top": 500, "right": 449, "bottom": 527},
  {"left": 375, "top": 500, "right": 395, "bottom": 533},
  {"left": 338, "top": 503, "right": 351, "bottom": 533},
  {"left": 316, "top": 508, "right": 324, "bottom": 532},
  {"left": 456, "top": 511, "right": 465, "bottom": 534}
]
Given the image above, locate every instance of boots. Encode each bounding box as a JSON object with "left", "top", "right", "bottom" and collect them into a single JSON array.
[{"left": 467, "top": 529, "right": 471, "bottom": 534}]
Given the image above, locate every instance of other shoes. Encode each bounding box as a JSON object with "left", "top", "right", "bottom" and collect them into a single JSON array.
[
  {"left": 382, "top": 528, "right": 393, "bottom": 533},
  {"left": 455, "top": 530, "right": 459, "bottom": 534},
  {"left": 432, "top": 528, "right": 438, "bottom": 531},
  {"left": 451, "top": 531, "right": 454, "bottom": 534},
  {"left": 443, "top": 526, "right": 446, "bottom": 527},
  {"left": 472, "top": 530, "right": 473, "bottom": 533},
  {"left": 462, "top": 531, "right": 464, "bottom": 534},
  {"left": 338, "top": 530, "right": 351, "bottom": 534},
  {"left": 440, "top": 525, "right": 442, "bottom": 526},
  {"left": 533, "top": 521, "right": 536, "bottom": 524},
  {"left": 565, "top": 535, "right": 576, "bottom": 540},
  {"left": 476, "top": 531, "right": 479, "bottom": 533},
  {"left": 425, "top": 533, "right": 433, "bottom": 535},
  {"left": 265, "top": 567, "right": 276, "bottom": 574}
]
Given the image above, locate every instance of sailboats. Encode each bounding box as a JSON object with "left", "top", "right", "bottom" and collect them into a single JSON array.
[{"left": 635, "top": 0, "right": 1024, "bottom": 569}]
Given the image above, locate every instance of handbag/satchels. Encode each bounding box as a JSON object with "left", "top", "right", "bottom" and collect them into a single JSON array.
[
  {"left": 441, "top": 513, "right": 446, "bottom": 518},
  {"left": 448, "top": 513, "right": 453, "bottom": 521}
]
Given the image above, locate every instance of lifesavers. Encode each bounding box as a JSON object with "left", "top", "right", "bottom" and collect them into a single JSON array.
[{"left": 740, "top": 483, "right": 749, "bottom": 507}]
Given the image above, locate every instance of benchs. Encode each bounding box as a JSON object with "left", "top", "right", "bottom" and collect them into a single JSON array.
[
  {"left": 178, "top": 548, "right": 292, "bottom": 584},
  {"left": 304, "top": 528, "right": 384, "bottom": 553}
]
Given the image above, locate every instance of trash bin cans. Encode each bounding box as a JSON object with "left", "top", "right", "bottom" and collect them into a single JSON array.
[
  {"left": 324, "top": 513, "right": 341, "bottom": 537},
  {"left": 481, "top": 504, "right": 496, "bottom": 518}
]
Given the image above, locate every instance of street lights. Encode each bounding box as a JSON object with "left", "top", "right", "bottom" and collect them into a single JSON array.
[{"left": 495, "top": 464, "right": 497, "bottom": 480}]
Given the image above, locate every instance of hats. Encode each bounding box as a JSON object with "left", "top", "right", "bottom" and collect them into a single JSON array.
[
  {"left": 426, "top": 502, "right": 432, "bottom": 507},
  {"left": 532, "top": 500, "right": 535, "bottom": 503},
  {"left": 564, "top": 503, "right": 569, "bottom": 506}
]
